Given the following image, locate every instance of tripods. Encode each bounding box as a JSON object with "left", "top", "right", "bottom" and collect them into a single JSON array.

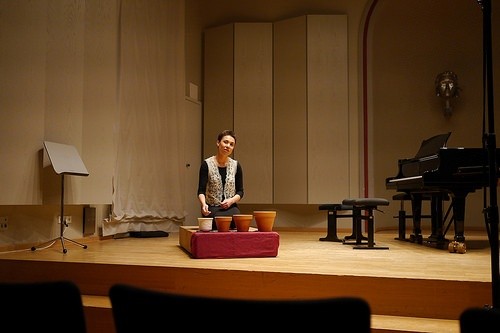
[{"left": 31, "top": 141, "right": 90, "bottom": 253}]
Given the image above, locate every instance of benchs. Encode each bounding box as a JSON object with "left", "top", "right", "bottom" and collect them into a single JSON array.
[
  {"left": 319, "top": 198, "right": 390, "bottom": 249},
  {"left": 393, "top": 192, "right": 450, "bottom": 239}
]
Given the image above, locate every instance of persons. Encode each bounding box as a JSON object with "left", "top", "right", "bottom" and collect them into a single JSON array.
[{"left": 197, "top": 130, "right": 244, "bottom": 230}]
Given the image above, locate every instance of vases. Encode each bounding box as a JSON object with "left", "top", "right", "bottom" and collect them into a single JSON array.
[
  {"left": 253, "top": 211, "right": 277, "bottom": 232},
  {"left": 215, "top": 216, "right": 232, "bottom": 232},
  {"left": 233, "top": 214, "right": 253, "bottom": 232},
  {"left": 198, "top": 218, "right": 213, "bottom": 232}
]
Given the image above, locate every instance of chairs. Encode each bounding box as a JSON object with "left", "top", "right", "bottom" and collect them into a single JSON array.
[
  {"left": 108, "top": 282, "right": 372, "bottom": 333},
  {"left": 0, "top": 279, "right": 88, "bottom": 333}
]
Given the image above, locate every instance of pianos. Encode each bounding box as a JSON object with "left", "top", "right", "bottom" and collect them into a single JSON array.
[{"left": 385, "top": 130, "right": 500, "bottom": 254}]
